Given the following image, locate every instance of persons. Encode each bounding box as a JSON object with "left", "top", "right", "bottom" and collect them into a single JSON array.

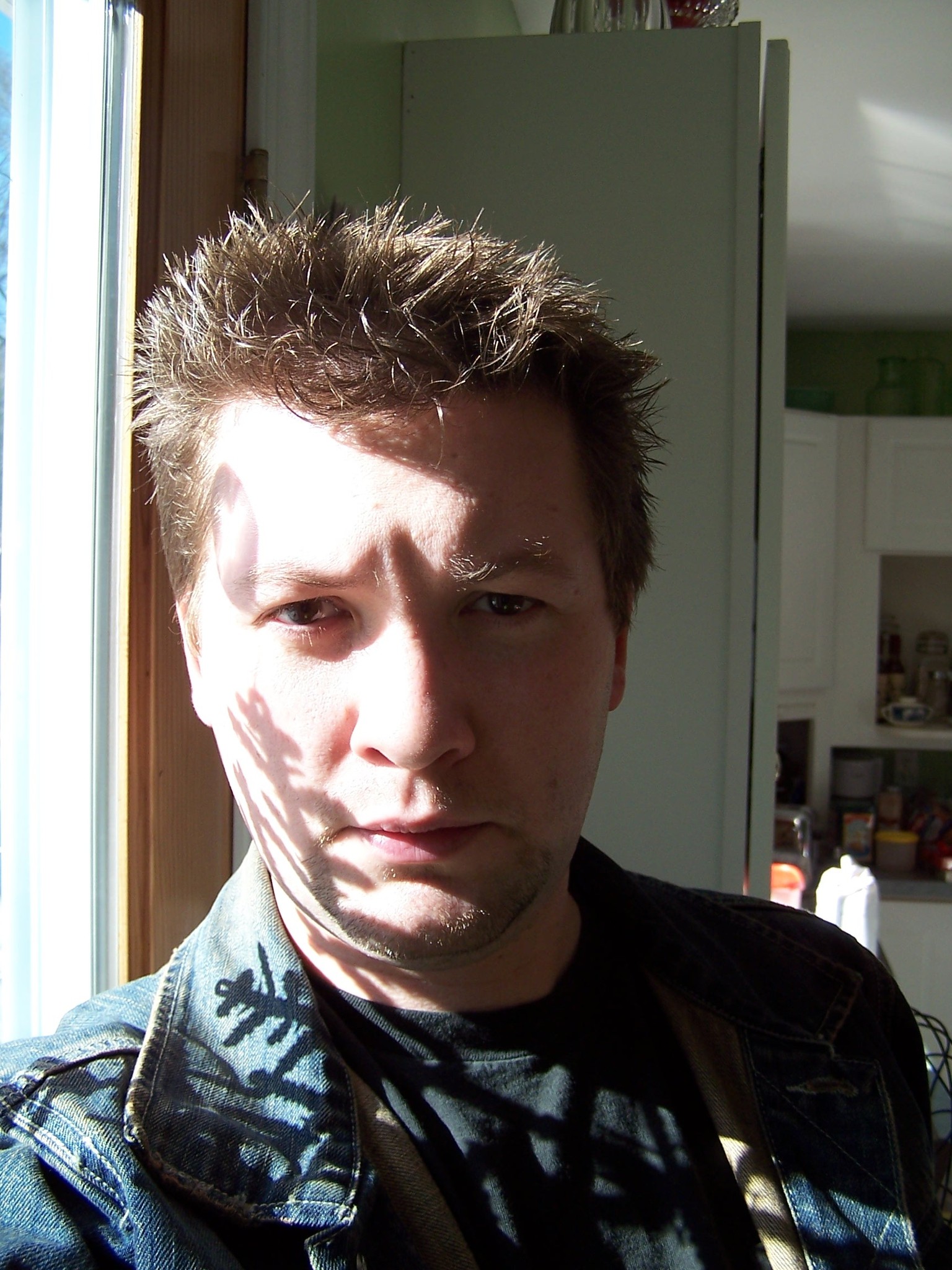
[{"left": 0, "top": 188, "right": 940, "bottom": 1270}]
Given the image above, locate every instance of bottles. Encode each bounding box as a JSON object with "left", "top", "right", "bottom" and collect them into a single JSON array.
[
  {"left": 742, "top": 861, "right": 806, "bottom": 909},
  {"left": 878, "top": 614, "right": 910, "bottom": 722},
  {"left": 914, "top": 631, "right": 952, "bottom": 718},
  {"left": 815, "top": 852, "right": 880, "bottom": 959},
  {"left": 881, "top": 696, "right": 932, "bottom": 727}
]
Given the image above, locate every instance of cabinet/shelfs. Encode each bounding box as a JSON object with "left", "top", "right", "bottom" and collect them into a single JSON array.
[
  {"left": 400, "top": 22, "right": 790, "bottom": 899},
  {"left": 773, "top": 410, "right": 952, "bottom": 1138}
]
